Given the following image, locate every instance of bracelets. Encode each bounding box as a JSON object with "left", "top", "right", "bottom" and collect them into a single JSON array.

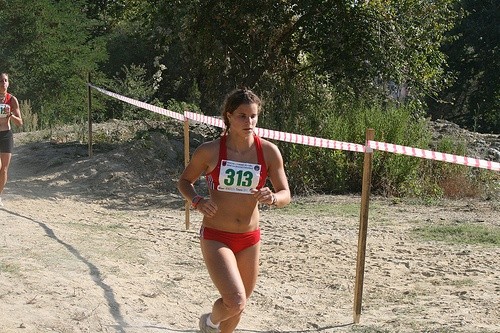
[
  {"left": 267, "top": 191, "right": 278, "bottom": 207},
  {"left": 6, "top": 111, "right": 13, "bottom": 118},
  {"left": 190, "top": 195, "right": 204, "bottom": 210}
]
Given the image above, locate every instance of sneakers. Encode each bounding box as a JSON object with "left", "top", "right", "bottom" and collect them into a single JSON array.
[{"left": 198, "top": 312, "right": 221, "bottom": 333}]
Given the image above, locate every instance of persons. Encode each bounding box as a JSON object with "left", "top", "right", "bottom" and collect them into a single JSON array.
[
  {"left": 176, "top": 86, "right": 293, "bottom": 332},
  {"left": 0, "top": 72, "right": 24, "bottom": 209}
]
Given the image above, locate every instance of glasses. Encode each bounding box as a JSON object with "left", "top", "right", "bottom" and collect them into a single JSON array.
[{"left": 0, "top": 78, "right": 8, "bottom": 82}]
